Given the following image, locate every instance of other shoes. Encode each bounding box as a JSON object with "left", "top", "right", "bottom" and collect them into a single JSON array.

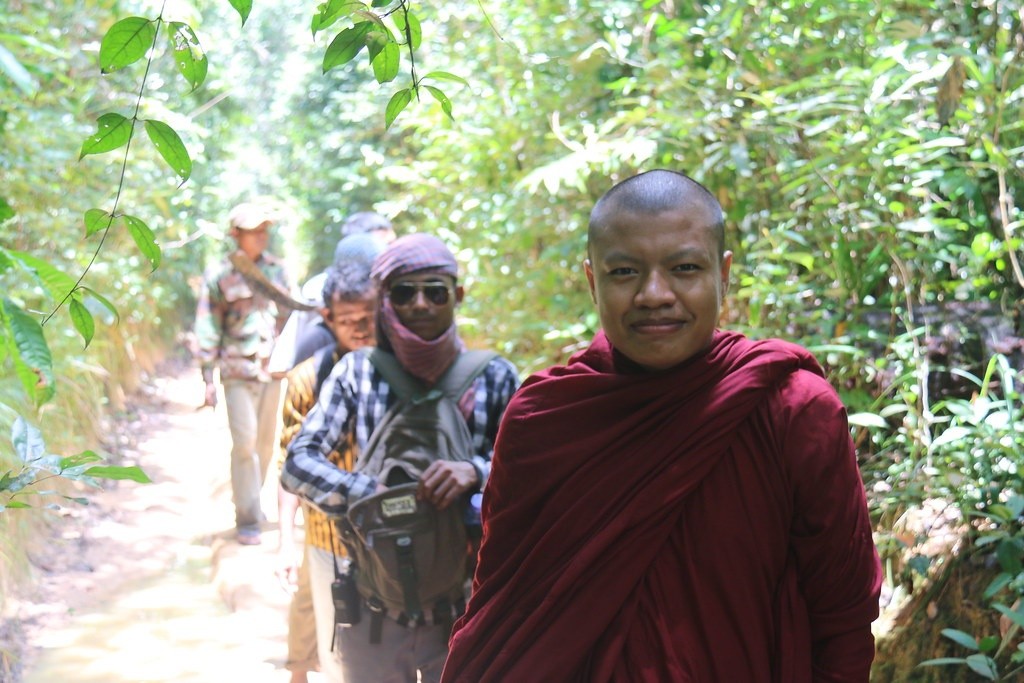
[
  {"left": 260, "top": 518, "right": 278, "bottom": 532},
  {"left": 237, "top": 527, "right": 260, "bottom": 545}
]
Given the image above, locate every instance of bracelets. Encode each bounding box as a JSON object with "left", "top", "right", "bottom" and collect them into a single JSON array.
[{"left": 463, "top": 455, "right": 489, "bottom": 491}]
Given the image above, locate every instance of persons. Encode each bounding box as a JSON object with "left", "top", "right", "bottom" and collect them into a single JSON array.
[
  {"left": 195, "top": 200, "right": 300, "bottom": 546},
  {"left": 279, "top": 235, "right": 528, "bottom": 683},
  {"left": 268, "top": 212, "right": 400, "bottom": 683},
  {"left": 439, "top": 169, "right": 884, "bottom": 683}
]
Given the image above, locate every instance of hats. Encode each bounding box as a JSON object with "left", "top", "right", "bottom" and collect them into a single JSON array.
[{"left": 228, "top": 202, "right": 274, "bottom": 230}]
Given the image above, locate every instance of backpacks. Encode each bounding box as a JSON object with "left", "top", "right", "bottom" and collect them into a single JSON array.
[{"left": 335, "top": 347, "right": 501, "bottom": 611}]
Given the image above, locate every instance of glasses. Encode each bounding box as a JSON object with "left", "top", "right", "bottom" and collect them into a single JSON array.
[{"left": 389, "top": 282, "right": 455, "bottom": 306}]
